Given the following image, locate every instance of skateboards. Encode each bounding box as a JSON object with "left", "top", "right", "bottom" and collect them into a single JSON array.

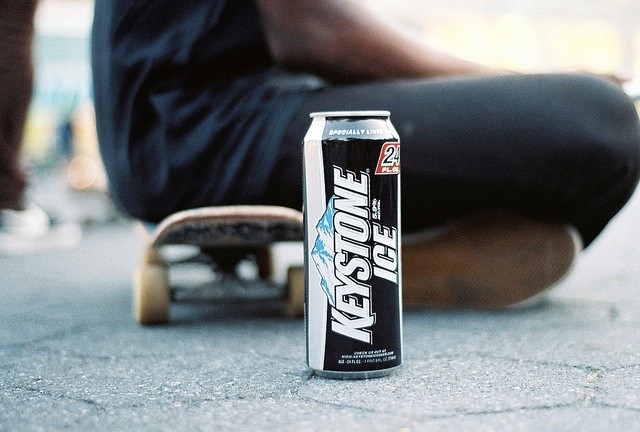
[{"left": 133, "top": 205, "right": 305, "bottom": 324}]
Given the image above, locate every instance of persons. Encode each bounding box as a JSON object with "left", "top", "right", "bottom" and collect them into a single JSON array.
[
  {"left": 91, "top": 0, "right": 640, "bottom": 311},
  {"left": 0, "top": 0, "right": 53, "bottom": 239}
]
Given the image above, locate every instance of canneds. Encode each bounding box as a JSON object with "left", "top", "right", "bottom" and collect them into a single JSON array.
[{"left": 300, "top": 109, "right": 404, "bottom": 379}]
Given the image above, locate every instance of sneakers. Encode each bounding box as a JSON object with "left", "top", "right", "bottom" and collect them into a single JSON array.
[{"left": 403, "top": 216, "right": 583, "bottom": 310}]
